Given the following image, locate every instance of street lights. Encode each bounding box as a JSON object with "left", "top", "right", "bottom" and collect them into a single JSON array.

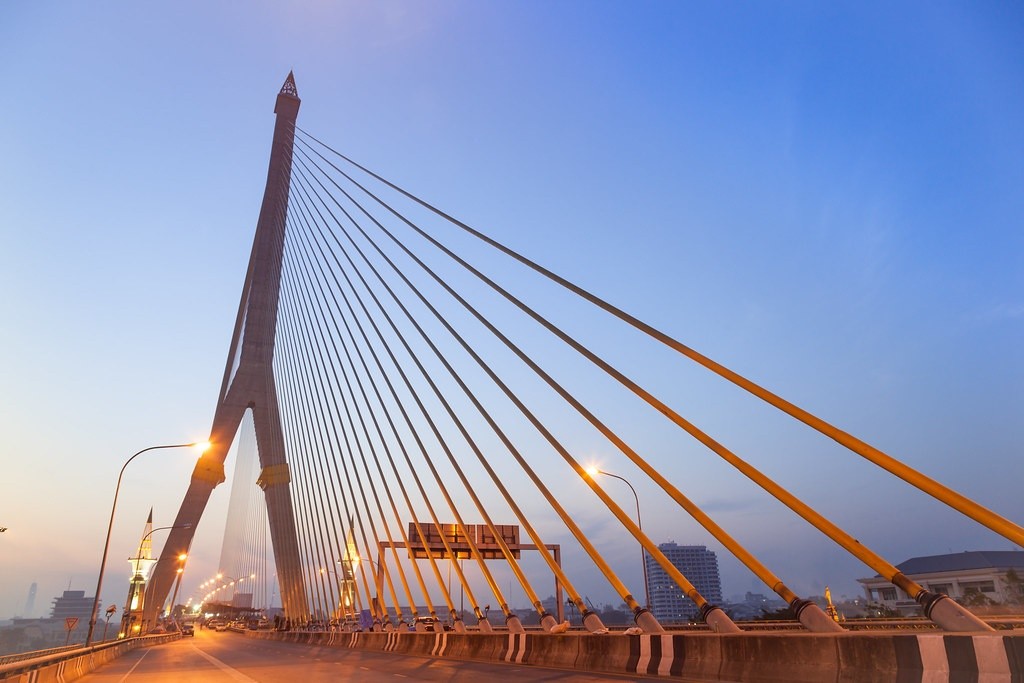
[
  {"left": 85, "top": 440, "right": 212, "bottom": 647},
  {"left": 143, "top": 554, "right": 188, "bottom": 631},
  {"left": 123, "top": 522, "right": 193, "bottom": 640},
  {"left": 337, "top": 558, "right": 378, "bottom": 566},
  {"left": 586, "top": 466, "right": 651, "bottom": 609},
  {"left": 318, "top": 569, "right": 340, "bottom": 579}
]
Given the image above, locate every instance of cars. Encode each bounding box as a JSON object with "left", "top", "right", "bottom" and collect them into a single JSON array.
[
  {"left": 216, "top": 623, "right": 226, "bottom": 631},
  {"left": 208, "top": 619, "right": 219, "bottom": 629},
  {"left": 181, "top": 624, "right": 194, "bottom": 636}
]
